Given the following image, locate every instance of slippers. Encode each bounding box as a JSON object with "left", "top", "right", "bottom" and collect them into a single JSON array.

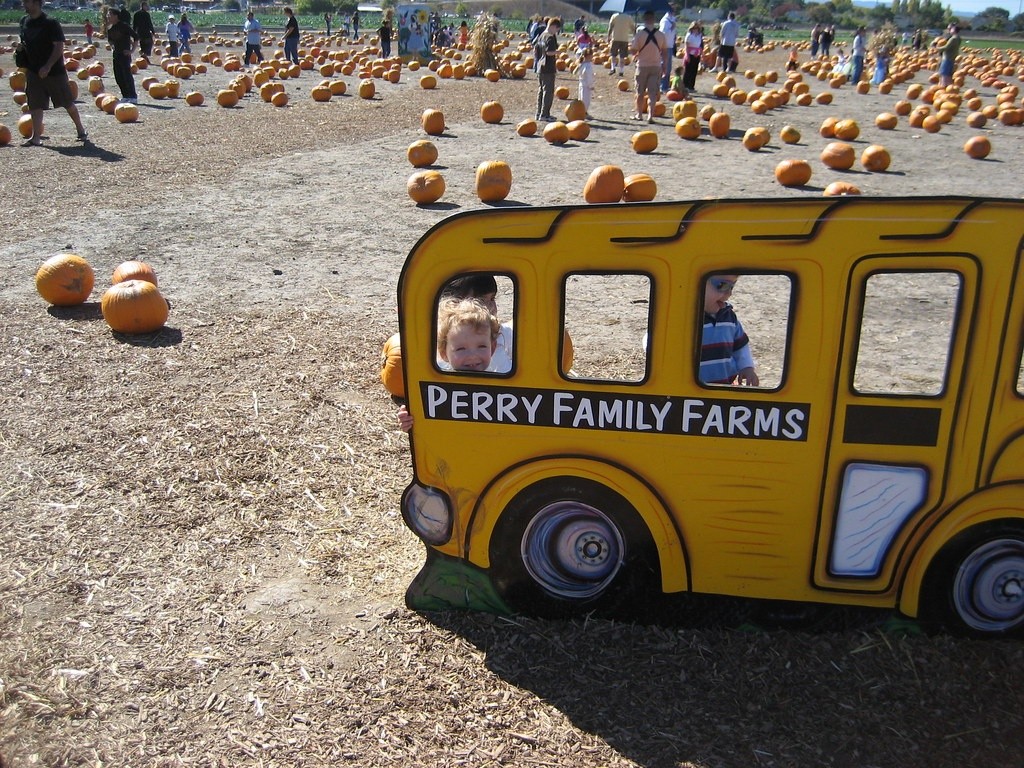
[
  {"left": 18, "top": 139, "right": 43, "bottom": 147},
  {"left": 76, "top": 131, "right": 88, "bottom": 141}
]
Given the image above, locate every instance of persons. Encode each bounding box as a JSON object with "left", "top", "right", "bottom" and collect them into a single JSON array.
[
  {"left": 643, "top": 274, "right": 759, "bottom": 387},
  {"left": 630, "top": 12, "right": 667, "bottom": 124},
  {"left": 786, "top": 45, "right": 797, "bottom": 73},
  {"left": 84, "top": 19, "right": 94, "bottom": 44},
  {"left": 351, "top": 11, "right": 359, "bottom": 40},
  {"left": 165, "top": 12, "right": 194, "bottom": 57},
  {"left": 106, "top": 2, "right": 156, "bottom": 103},
  {"left": 375, "top": 20, "right": 393, "bottom": 58},
  {"left": 526, "top": 13, "right": 596, "bottom": 120},
  {"left": 659, "top": 3, "right": 739, "bottom": 99},
  {"left": 344, "top": 11, "right": 351, "bottom": 36},
  {"left": 430, "top": 12, "right": 470, "bottom": 48},
  {"left": 324, "top": 12, "right": 332, "bottom": 36},
  {"left": 242, "top": 11, "right": 265, "bottom": 67},
  {"left": 607, "top": 13, "right": 636, "bottom": 76},
  {"left": 280, "top": 7, "right": 300, "bottom": 66},
  {"left": 396, "top": 274, "right": 513, "bottom": 433},
  {"left": 13, "top": 0, "right": 88, "bottom": 146},
  {"left": 746, "top": 23, "right": 762, "bottom": 47},
  {"left": 810, "top": 24, "right": 835, "bottom": 60},
  {"left": 850, "top": 22, "right": 962, "bottom": 88}
]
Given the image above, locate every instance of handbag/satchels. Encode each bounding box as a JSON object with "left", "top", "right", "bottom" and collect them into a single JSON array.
[{"left": 14, "top": 43, "right": 27, "bottom": 68}]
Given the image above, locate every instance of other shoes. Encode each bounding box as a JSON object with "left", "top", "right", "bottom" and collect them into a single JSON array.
[
  {"left": 242, "top": 64, "right": 249, "bottom": 67},
  {"left": 708, "top": 67, "right": 718, "bottom": 72}
]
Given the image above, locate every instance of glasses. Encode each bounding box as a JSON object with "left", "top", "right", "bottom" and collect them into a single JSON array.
[{"left": 710, "top": 276, "right": 736, "bottom": 291}]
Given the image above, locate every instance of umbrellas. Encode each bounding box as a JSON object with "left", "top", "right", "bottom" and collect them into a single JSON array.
[{"left": 598, "top": 0, "right": 671, "bottom": 27}]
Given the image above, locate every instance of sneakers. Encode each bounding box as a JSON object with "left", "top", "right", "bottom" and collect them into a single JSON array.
[{"left": 534, "top": 113, "right": 557, "bottom": 121}]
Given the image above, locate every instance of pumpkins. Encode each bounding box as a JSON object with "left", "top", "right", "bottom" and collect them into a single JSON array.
[
  {"left": 0, "top": 32, "right": 1024, "bottom": 166},
  {"left": 379, "top": 333, "right": 404, "bottom": 397},
  {"left": 563, "top": 331, "right": 575, "bottom": 374},
  {"left": 622, "top": 174, "right": 657, "bottom": 201},
  {"left": 101, "top": 280, "right": 169, "bottom": 334},
  {"left": 111, "top": 261, "right": 159, "bottom": 288},
  {"left": 35, "top": 254, "right": 94, "bottom": 306},
  {"left": 584, "top": 164, "right": 624, "bottom": 203},
  {"left": 775, "top": 159, "right": 812, "bottom": 186},
  {"left": 407, "top": 170, "right": 445, "bottom": 205},
  {"left": 476, "top": 160, "right": 512, "bottom": 202},
  {"left": 823, "top": 182, "right": 867, "bottom": 197}
]
